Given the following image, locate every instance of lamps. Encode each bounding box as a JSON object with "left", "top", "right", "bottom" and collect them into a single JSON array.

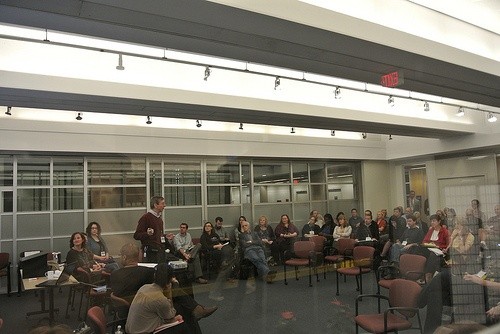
[
  {"left": 424, "top": 102, "right": 429, "bottom": 111},
  {"left": 6, "top": 54, "right": 395, "bottom": 140},
  {"left": 487, "top": 112, "right": 497, "bottom": 122},
  {"left": 458, "top": 107, "right": 464, "bottom": 117}
]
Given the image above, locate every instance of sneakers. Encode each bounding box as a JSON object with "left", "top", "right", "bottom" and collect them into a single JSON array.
[{"left": 192, "top": 305, "right": 218, "bottom": 321}]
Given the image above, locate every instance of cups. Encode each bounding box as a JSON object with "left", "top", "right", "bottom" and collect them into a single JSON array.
[
  {"left": 45, "top": 271, "right": 53, "bottom": 280},
  {"left": 310, "top": 231, "right": 314, "bottom": 235},
  {"left": 366, "top": 237, "right": 370, "bottom": 240}
]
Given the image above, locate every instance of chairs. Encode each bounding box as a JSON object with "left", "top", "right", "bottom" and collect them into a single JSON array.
[
  {"left": 0, "top": 253, "right": 12, "bottom": 297},
  {"left": 284, "top": 236, "right": 426, "bottom": 334},
  {"left": 66, "top": 266, "right": 130, "bottom": 334}
]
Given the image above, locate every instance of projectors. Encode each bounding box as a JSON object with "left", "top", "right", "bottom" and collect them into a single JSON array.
[{"left": 169, "top": 260, "right": 188, "bottom": 270}]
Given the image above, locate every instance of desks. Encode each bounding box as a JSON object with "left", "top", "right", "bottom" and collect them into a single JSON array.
[{"left": 20, "top": 269, "right": 78, "bottom": 327}]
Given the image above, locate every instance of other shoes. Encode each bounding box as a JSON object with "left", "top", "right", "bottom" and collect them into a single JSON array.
[
  {"left": 195, "top": 277, "right": 209, "bottom": 284},
  {"left": 384, "top": 275, "right": 395, "bottom": 279}
]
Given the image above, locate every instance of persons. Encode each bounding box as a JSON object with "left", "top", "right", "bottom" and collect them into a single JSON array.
[
  {"left": 275, "top": 214, "right": 300, "bottom": 255},
  {"left": 234, "top": 216, "right": 247, "bottom": 268},
  {"left": 310, "top": 208, "right": 325, "bottom": 228},
  {"left": 238, "top": 221, "right": 271, "bottom": 275},
  {"left": 133, "top": 197, "right": 176, "bottom": 262},
  {"left": 173, "top": 222, "right": 208, "bottom": 284},
  {"left": 105, "top": 242, "right": 217, "bottom": 334},
  {"left": 332, "top": 191, "right": 500, "bottom": 334},
  {"left": 65, "top": 222, "right": 120, "bottom": 286},
  {"left": 199, "top": 222, "right": 223, "bottom": 280},
  {"left": 318, "top": 214, "right": 338, "bottom": 247},
  {"left": 254, "top": 216, "right": 277, "bottom": 266},
  {"left": 213, "top": 217, "right": 230, "bottom": 266},
  {"left": 464, "top": 272, "right": 500, "bottom": 319},
  {"left": 301, "top": 216, "right": 321, "bottom": 240}
]
